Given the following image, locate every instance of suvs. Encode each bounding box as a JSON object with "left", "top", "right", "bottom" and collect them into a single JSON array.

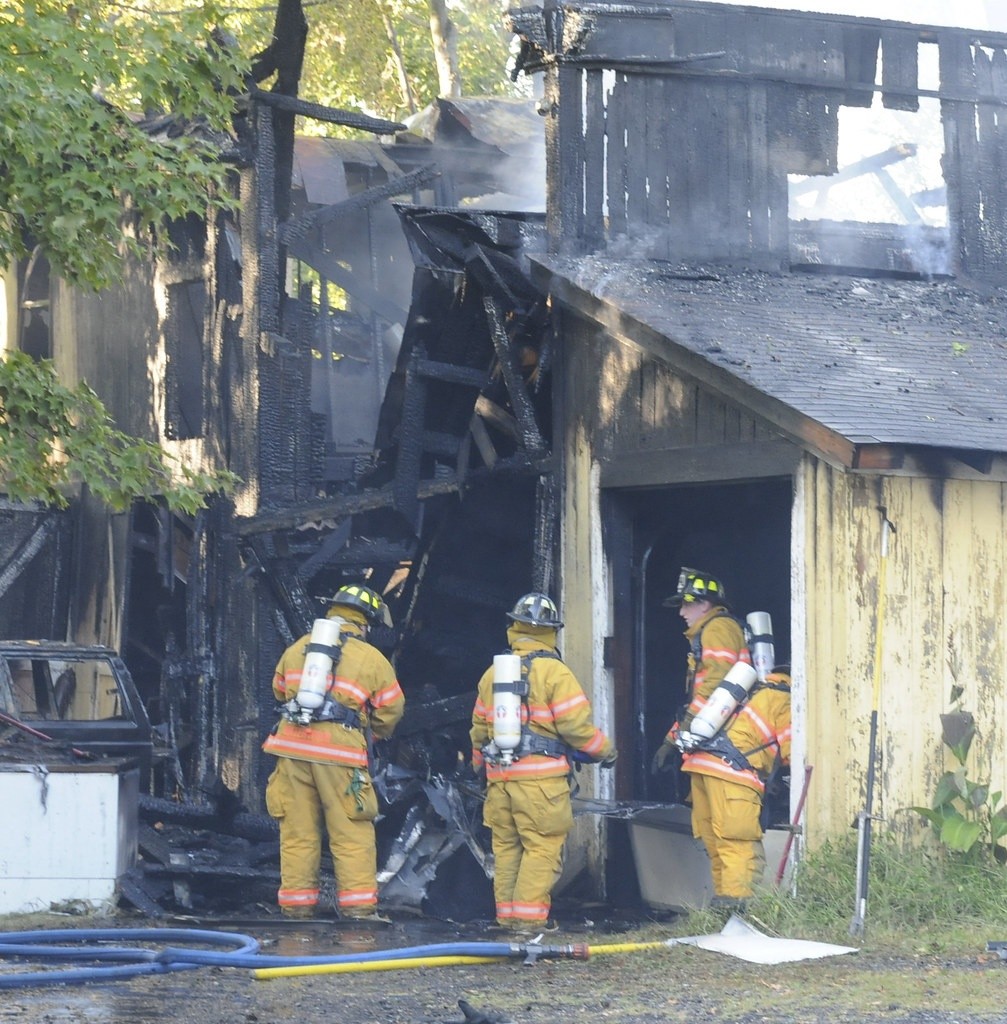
[{"left": 1, "top": 639, "right": 155, "bottom": 772}]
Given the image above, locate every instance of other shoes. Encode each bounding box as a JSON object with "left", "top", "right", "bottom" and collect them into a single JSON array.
[
  {"left": 341, "top": 907, "right": 372, "bottom": 916},
  {"left": 281, "top": 906, "right": 313, "bottom": 918},
  {"left": 501, "top": 920, "right": 558, "bottom": 932}
]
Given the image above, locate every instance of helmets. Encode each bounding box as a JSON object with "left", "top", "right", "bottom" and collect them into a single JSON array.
[
  {"left": 662, "top": 568, "right": 733, "bottom": 610},
  {"left": 315, "top": 585, "right": 385, "bottom": 626},
  {"left": 506, "top": 593, "right": 563, "bottom": 627}
]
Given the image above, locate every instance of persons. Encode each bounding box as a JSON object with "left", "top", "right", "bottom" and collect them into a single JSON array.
[
  {"left": 262, "top": 583, "right": 406, "bottom": 930},
  {"left": 470, "top": 592, "right": 617, "bottom": 932},
  {"left": 648, "top": 566, "right": 753, "bottom": 778},
  {"left": 680, "top": 662, "right": 793, "bottom": 917}
]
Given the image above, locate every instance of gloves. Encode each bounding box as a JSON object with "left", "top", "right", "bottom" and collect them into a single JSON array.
[
  {"left": 651, "top": 739, "right": 677, "bottom": 773},
  {"left": 601, "top": 748, "right": 618, "bottom": 769}
]
